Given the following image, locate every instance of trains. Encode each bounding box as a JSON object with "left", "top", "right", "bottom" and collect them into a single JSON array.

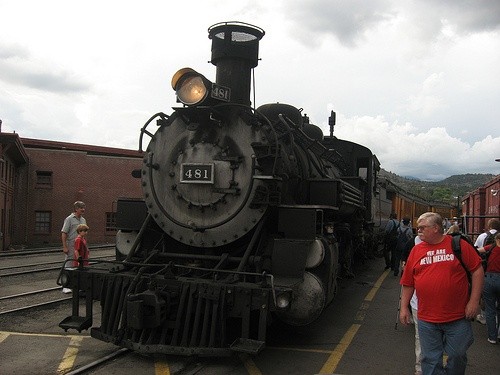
[
  {"left": 456, "top": 174, "right": 500, "bottom": 248},
  {"left": 52, "top": 20, "right": 455, "bottom": 361}
]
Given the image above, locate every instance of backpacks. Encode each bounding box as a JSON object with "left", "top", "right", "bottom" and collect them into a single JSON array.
[
  {"left": 448, "top": 232, "right": 480, "bottom": 283},
  {"left": 388, "top": 219, "right": 400, "bottom": 242},
  {"left": 398, "top": 228, "right": 410, "bottom": 248},
  {"left": 482, "top": 231, "right": 499, "bottom": 246}
]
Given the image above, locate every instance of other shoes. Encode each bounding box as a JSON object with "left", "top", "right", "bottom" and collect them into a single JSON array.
[
  {"left": 476, "top": 314, "right": 486, "bottom": 324},
  {"left": 487, "top": 336, "right": 497, "bottom": 344},
  {"left": 62, "top": 288, "right": 72, "bottom": 294}
]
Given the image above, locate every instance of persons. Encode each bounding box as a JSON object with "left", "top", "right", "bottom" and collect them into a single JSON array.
[
  {"left": 381, "top": 212, "right": 459, "bottom": 278},
  {"left": 473, "top": 219, "right": 500, "bottom": 343},
  {"left": 74, "top": 224, "right": 90, "bottom": 267},
  {"left": 398, "top": 212, "right": 485, "bottom": 375},
  {"left": 410, "top": 289, "right": 422, "bottom": 375},
  {"left": 61, "top": 200, "right": 87, "bottom": 293}
]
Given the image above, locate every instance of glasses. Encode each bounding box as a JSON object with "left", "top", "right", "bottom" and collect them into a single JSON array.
[{"left": 416, "top": 225, "right": 436, "bottom": 230}]
